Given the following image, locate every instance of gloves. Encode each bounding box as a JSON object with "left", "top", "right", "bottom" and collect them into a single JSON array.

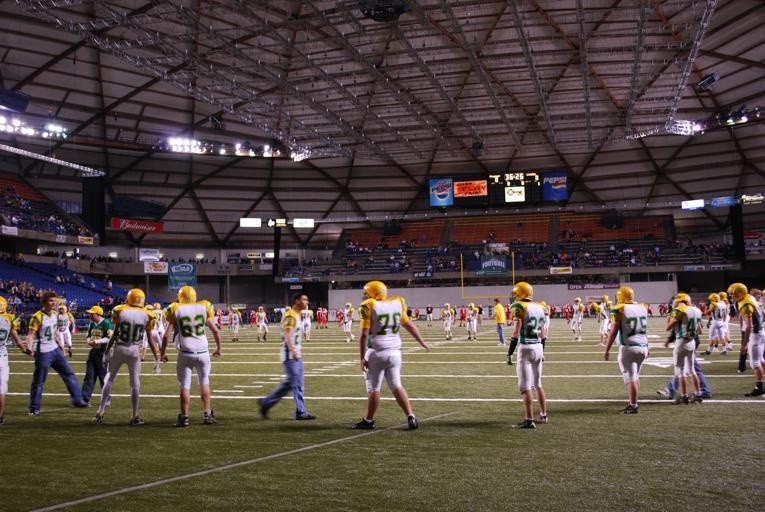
[
  {"left": 506, "top": 355, "right": 513, "bottom": 366},
  {"left": 152, "top": 359, "right": 162, "bottom": 375},
  {"left": 102, "top": 351, "right": 111, "bottom": 369}
]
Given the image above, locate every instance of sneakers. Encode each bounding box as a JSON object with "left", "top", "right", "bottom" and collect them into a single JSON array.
[
  {"left": 446, "top": 335, "right": 476, "bottom": 341},
  {"left": 1, "top": 416, "right": 7, "bottom": 425},
  {"left": 700, "top": 342, "right": 734, "bottom": 356},
  {"left": 175, "top": 414, "right": 190, "bottom": 426},
  {"left": 257, "top": 398, "right": 272, "bottom": 420},
  {"left": 72, "top": 399, "right": 92, "bottom": 408},
  {"left": 540, "top": 411, "right": 549, "bottom": 424},
  {"left": 203, "top": 411, "right": 218, "bottom": 425},
  {"left": 305, "top": 323, "right": 355, "bottom": 343},
  {"left": 408, "top": 416, "right": 419, "bottom": 430},
  {"left": 744, "top": 387, "right": 765, "bottom": 398},
  {"left": 351, "top": 418, "right": 377, "bottom": 430},
  {"left": 91, "top": 414, "right": 103, "bottom": 423},
  {"left": 573, "top": 333, "right": 609, "bottom": 347},
  {"left": 736, "top": 362, "right": 748, "bottom": 374},
  {"left": 104, "top": 396, "right": 112, "bottom": 407},
  {"left": 656, "top": 386, "right": 713, "bottom": 405},
  {"left": 295, "top": 412, "right": 318, "bottom": 421},
  {"left": 231, "top": 337, "right": 268, "bottom": 343},
  {"left": 129, "top": 416, "right": 145, "bottom": 425},
  {"left": 511, "top": 418, "right": 536, "bottom": 429},
  {"left": 28, "top": 409, "right": 40, "bottom": 417},
  {"left": 616, "top": 404, "right": 640, "bottom": 415},
  {"left": 497, "top": 343, "right": 506, "bottom": 346}
]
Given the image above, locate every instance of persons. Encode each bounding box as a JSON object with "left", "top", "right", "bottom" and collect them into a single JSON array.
[
  {"left": 655, "top": 296, "right": 712, "bottom": 399},
  {"left": 0, "top": 295, "right": 29, "bottom": 425},
  {"left": 256, "top": 292, "right": 318, "bottom": 420},
  {"left": 26, "top": 291, "right": 90, "bottom": 417},
  {"left": 351, "top": 280, "right": 430, "bottom": 429},
  {"left": 160, "top": 286, "right": 222, "bottom": 427},
  {"left": 81, "top": 305, "right": 114, "bottom": 406},
  {"left": 507, "top": 282, "right": 551, "bottom": 428},
  {"left": 603, "top": 286, "right": 649, "bottom": 414},
  {"left": 2, "top": 182, "right": 765, "bottom": 351},
  {"left": 90, "top": 287, "right": 160, "bottom": 425},
  {"left": 727, "top": 283, "right": 765, "bottom": 397},
  {"left": 665, "top": 293, "right": 702, "bottom": 405}
]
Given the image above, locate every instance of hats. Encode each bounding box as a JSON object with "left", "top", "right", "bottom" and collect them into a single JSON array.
[{"left": 85, "top": 305, "right": 104, "bottom": 316}]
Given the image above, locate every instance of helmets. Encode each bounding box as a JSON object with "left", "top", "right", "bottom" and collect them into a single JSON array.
[
  {"left": 719, "top": 291, "right": 728, "bottom": 301},
  {"left": 58, "top": 305, "right": 68, "bottom": 313},
  {"left": 345, "top": 303, "right": 352, "bottom": 308},
  {"left": 511, "top": 281, "right": 534, "bottom": 301},
  {"left": 0, "top": 295, "right": 8, "bottom": 314},
  {"left": 674, "top": 293, "right": 692, "bottom": 305},
  {"left": 178, "top": 285, "right": 197, "bottom": 304},
  {"left": 615, "top": 286, "right": 636, "bottom": 304},
  {"left": 574, "top": 295, "right": 609, "bottom": 309},
  {"left": 708, "top": 293, "right": 720, "bottom": 302},
  {"left": 444, "top": 303, "right": 474, "bottom": 309},
  {"left": 145, "top": 303, "right": 161, "bottom": 310},
  {"left": 127, "top": 288, "right": 146, "bottom": 308},
  {"left": 363, "top": 281, "right": 388, "bottom": 300},
  {"left": 726, "top": 282, "right": 749, "bottom": 297}
]
